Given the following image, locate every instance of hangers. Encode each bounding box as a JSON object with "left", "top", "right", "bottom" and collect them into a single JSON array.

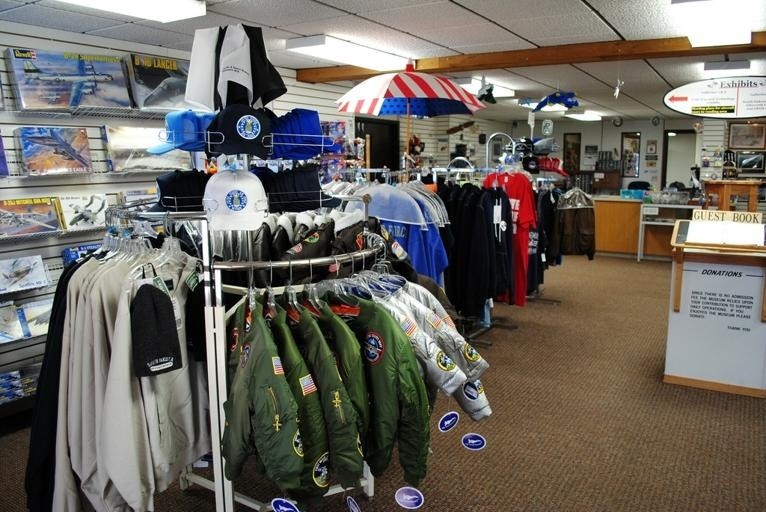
[
  {"left": 315, "top": 162, "right": 593, "bottom": 200},
  {"left": 92, "top": 203, "right": 199, "bottom": 285},
  {"left": 234, "top": 227, "right": 416, "bottom": 320}
]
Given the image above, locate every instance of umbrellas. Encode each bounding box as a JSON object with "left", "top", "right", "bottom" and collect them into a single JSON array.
[{"left": 335, "top": 65, "right": 487, "bottom": 183}]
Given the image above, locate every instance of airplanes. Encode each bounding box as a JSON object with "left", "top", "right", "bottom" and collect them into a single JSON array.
[
  {"left": 2, "top": 258, "right": 38, "bottom": 287},
  {"left": 0, "top": 205, "right": 57, "bottom": 231},
  {"left": 143, "top": 66, "right": 189, "bottom": 108},
  {"left": 23, "top": 54, "right": 115, "bottom": 107},
  {"left": 69, "top": 194, "right": 105, "bottom": 229},
  {"left": 26, "top": 128, "right": 91, "bottom": 169}
]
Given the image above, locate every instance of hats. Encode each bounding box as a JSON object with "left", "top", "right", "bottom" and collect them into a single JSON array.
[
  {"left": 145, "top": 109, "right": 218, "bottom": 153},
  {"left": 147, "top": 165, "right": 343, "bottom": 234},
  {"left": 205, "top": 104, "right": 272, "bottom": 161},
  {"left": 272, "top": 108, "right": 343, "bottom": 160}
]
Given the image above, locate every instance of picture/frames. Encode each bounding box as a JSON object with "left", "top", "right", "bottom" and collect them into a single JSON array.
[
  {"left": 735, "top": 149, "right": 766, "bottom": 174},
  {"left": 620, "top": 130, "right": 641, "bottom": 178},
  {"left": 562, "top": 131, "right": 581, "bottom": 175},
  {"left": 727, "top": 120, "right": 766, "bottom": 150}
]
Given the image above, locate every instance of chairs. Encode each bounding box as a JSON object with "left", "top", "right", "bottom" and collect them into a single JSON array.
[{"left": 627, "top": 180, "right": 652, "bottom": 190}]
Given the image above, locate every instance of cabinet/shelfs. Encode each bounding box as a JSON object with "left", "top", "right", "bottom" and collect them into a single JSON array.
[
  {"left": 0, "top": 100, "right": 197, "bottom": 410},
  {"left": 635, "top": 203, "right": 704, "bottom": 264}
]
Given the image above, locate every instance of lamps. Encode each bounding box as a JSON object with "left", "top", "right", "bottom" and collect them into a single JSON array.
[
  {"left": 612, "top": 48, "right": 625, "bottom": 99},
  {"left": 283, "top": 30, "right": 418, "bottom": 75},
  {"left": 51, "top": 0, "right": 211, "bottom": 26}
]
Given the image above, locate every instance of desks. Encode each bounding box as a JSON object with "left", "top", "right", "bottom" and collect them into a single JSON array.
[{"left": 700, "top": 177, "right": 764, "bottom": 214}]
